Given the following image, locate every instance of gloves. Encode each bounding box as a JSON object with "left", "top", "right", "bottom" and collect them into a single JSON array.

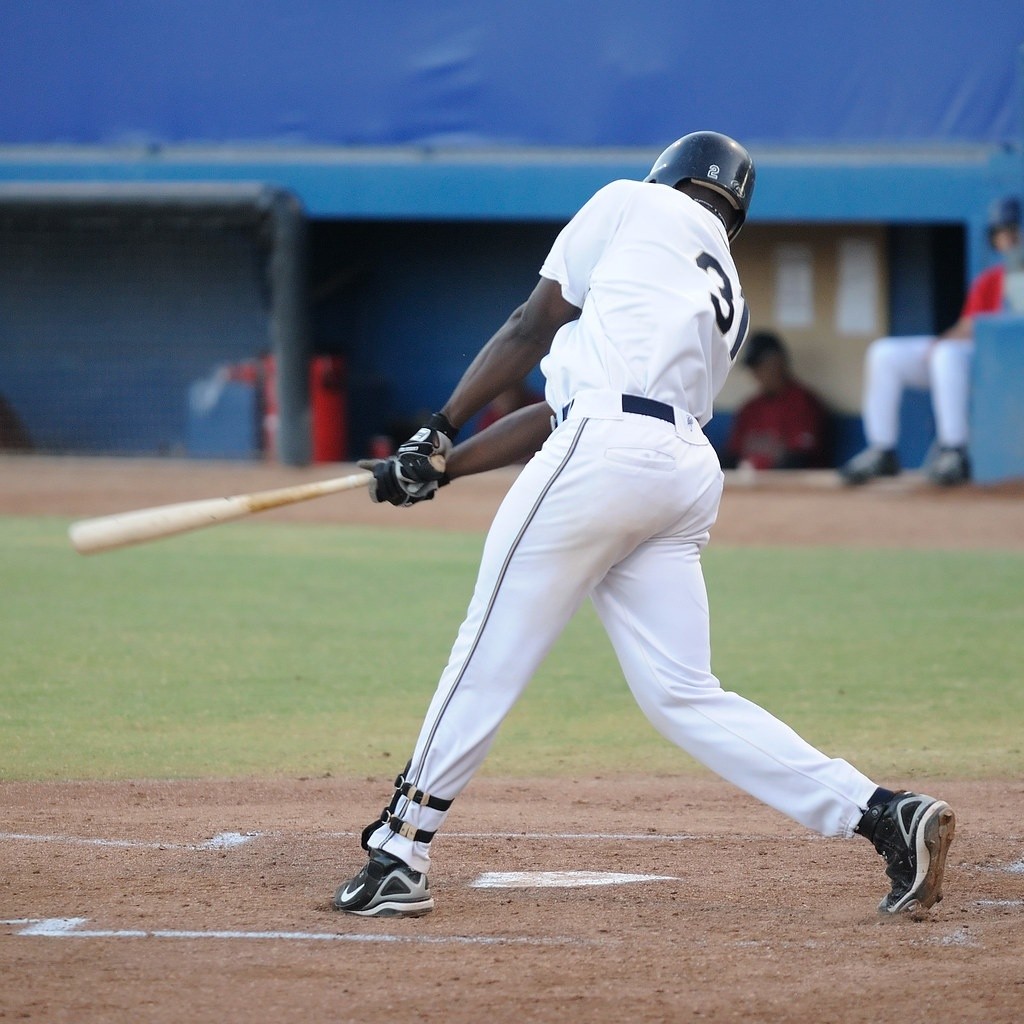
[
  {"left": 395, "top": 411, "right": 460, "bottom": 484},
  {"left": 356, "top": 455, "right": 450, "bottom": 507}
]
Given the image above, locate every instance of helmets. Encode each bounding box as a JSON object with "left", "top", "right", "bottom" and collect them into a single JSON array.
[
  {"left": 643, "top": 131, "right": 756, "bottom": 244},
  {"left": 984, "top": 195, "right": 1024, "bottom": 231}
]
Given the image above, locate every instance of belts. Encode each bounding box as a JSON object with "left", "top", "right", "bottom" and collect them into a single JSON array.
[{"left": 550, "top": 394, "right": 675, "bottom": 431}]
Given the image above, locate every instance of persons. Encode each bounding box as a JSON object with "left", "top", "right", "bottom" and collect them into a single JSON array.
[
  {"left": 333, "top": 132, "right": 956, "bottom": 920},
  {"left": 478, "top": 197, "right": 1023, "bottom": 491}
]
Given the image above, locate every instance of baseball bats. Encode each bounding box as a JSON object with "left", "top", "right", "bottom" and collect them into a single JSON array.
[{"left": 66, "top": 454, "right": 447, "bottom": 554}]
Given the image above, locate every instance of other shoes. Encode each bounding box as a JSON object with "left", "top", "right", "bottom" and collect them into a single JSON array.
[
  {"left": 930, "top": 446, "right": 970, "bottom": 486},
  {"left": 836, "top": 450, "right": 900, "bottom": 484}
]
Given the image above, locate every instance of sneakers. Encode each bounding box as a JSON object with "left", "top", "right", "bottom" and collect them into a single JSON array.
[
  {"left": 335, "top": 848, "right": 434, "bottom": 918},
  {"left": 854, "top": 791, "right": 955, "bottom": 914}
]
